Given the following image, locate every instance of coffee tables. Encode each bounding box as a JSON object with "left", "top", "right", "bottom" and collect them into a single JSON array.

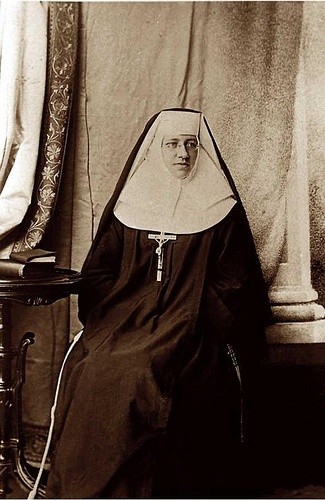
[{"left": 0, "top": 267, "right": 86, "bottom": 499}]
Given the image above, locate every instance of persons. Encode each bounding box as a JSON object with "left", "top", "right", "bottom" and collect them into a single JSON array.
[{"left": 46, "top": 108, "right": 268, "bottom": 500}]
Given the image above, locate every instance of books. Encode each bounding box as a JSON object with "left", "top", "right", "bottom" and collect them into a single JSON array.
[{"left": 0, "top": 249, "right": 57, "bottom": 279}]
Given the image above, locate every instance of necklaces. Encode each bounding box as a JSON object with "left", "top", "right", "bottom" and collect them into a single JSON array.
[{"left": 146, "top": 230, "right": 175, "bottom": 283}]
